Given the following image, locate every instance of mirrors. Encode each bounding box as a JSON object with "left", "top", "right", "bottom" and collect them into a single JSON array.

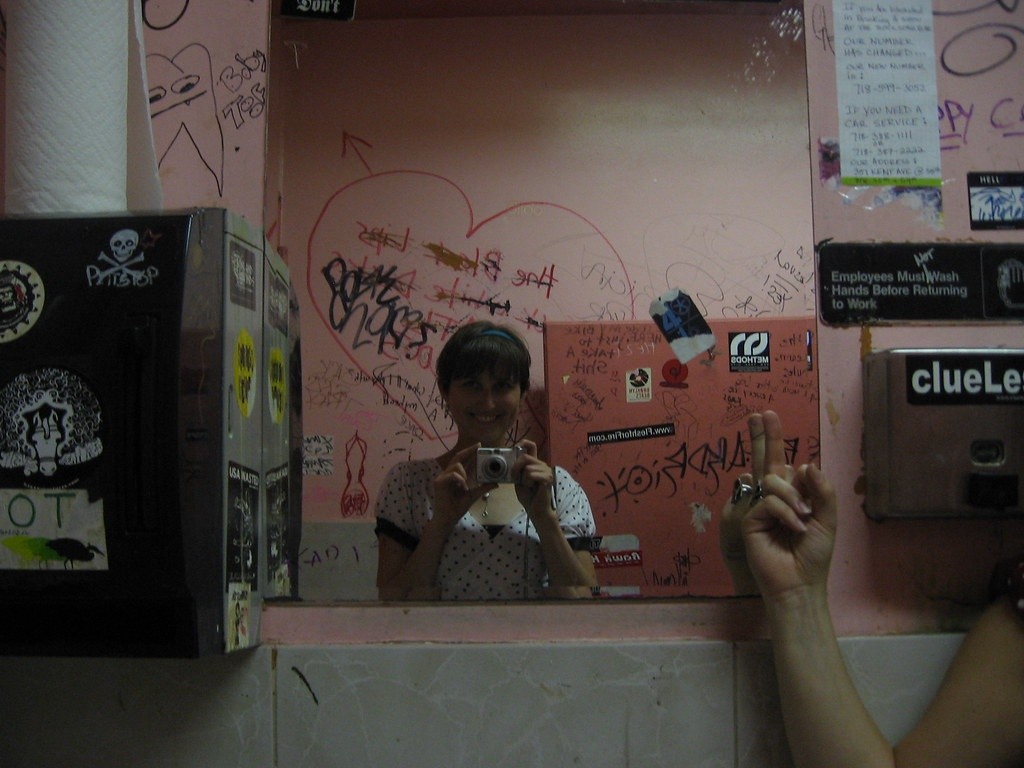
[{"left": 251, "top": 0, "right": 823, "bottom": 604}]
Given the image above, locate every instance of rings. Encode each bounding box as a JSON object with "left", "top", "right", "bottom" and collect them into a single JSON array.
[
  {"left": 731, "top": 477, "right": 752, "bottom": 505},
  {"left": 755, "top": 478, "right": 763, "bottom": 502}
]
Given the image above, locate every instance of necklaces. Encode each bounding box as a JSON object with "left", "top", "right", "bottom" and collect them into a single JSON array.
[{"left": 481, "top": 491, "right": 490, "bottom": 518}]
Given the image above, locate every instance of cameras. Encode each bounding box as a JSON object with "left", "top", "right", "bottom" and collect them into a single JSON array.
[{"left": 475, "top": 448, "right": 526, "bottom": 484}]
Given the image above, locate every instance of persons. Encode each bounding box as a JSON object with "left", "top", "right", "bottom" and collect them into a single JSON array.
[
  {"left": 739, "top": 410, "right": 1024, "bottom": 768},
  {"left": 717, "top": 413, "right": 766, "bottom": 600},
  {"left": 374, "top": 321, "right": 601, "bottom": 601}
]
